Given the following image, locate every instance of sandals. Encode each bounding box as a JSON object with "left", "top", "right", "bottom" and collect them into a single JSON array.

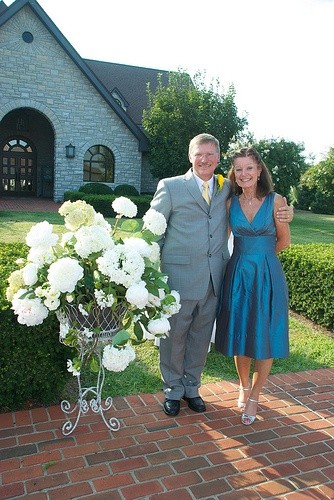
[
  {"left": 239, "top": 386, "right": 251, "bottom": 412},
  {"left": 242, "top": 398, "right": 258, "bottom": 425}
]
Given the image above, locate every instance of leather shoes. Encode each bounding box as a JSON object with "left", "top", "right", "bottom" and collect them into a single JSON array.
[
  {"left": 163, "top": 398, "right": 180, "bottom": 416},
  {"left": 183, "top": 396, "right": 206, "bottom": 412}
]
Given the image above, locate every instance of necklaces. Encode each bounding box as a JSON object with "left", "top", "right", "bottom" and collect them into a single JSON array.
[{"left": 241, "top": 192, "right": 260, "bottom": 206}]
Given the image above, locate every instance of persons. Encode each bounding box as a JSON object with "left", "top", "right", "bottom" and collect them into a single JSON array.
[
  {"left": 215, "top": 147, "right": 290, "bottom": 425},
  {"left": 142, "top": 133, "right": 293, "bottom": 416}
]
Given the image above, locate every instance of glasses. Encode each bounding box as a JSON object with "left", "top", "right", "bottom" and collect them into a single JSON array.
[{"left": 190, "top": 151, "right": 217, "bottom": 157}]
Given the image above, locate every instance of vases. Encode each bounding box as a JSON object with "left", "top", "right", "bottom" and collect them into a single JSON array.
[{"left": 54, "top": 302, "right": 128, "bottom": 344}]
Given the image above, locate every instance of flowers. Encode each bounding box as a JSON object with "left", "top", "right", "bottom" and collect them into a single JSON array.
[
  {"left": 216, "top": 173, "right": 225, "bottom": 193},
  {"left": 5, "top": 197, "right": 181, "bottom": 375}
]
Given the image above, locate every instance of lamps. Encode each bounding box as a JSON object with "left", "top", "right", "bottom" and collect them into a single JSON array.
[{"left": 66, "top": 143, "right": 75, "bottom": 158}]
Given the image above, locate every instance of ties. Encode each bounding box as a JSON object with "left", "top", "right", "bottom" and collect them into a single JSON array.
[{"left": 202, "top": 182, "right": 211, "bottom": 205}]
